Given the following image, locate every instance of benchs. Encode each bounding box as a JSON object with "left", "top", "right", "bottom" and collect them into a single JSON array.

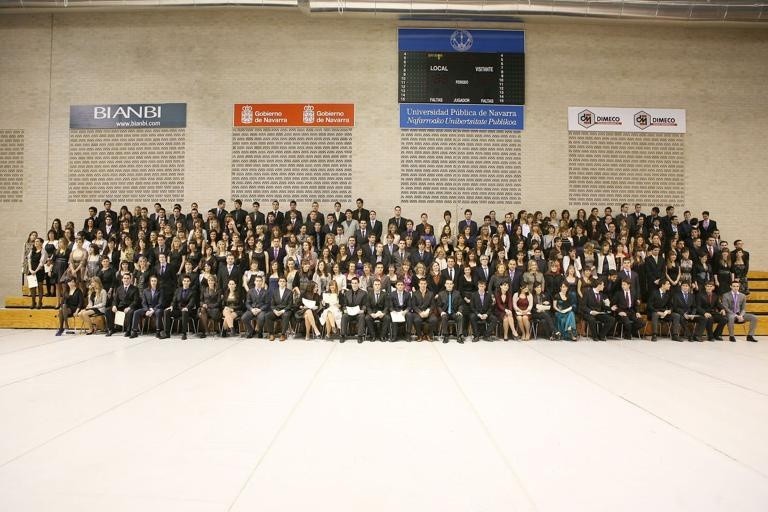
[
  {"left": 0, "top": 283, "right": 108, "bottom": 328},
  {"left": 638, "top": 270, "right": 768, "bottom": 336}
]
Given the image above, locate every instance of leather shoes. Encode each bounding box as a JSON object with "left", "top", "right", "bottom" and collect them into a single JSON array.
[
  {"left": 549, "top": 328, "right": 735, "bottom": 342},
  {"left": 55, "top": 329, "right": 530, "bottom": 343}
]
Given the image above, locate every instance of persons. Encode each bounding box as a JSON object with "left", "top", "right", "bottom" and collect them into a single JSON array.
[{"left": 22, "top": 197, "right": 761, "bottom": 344}]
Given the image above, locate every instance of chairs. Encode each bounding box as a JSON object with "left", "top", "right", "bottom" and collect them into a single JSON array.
[{"left": 53, "top": 290, "right": 750, "bottom": 340}]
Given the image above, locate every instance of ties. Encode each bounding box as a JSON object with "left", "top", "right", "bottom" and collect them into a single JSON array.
[
  {"left": 733, "top": 294, "right": 737, "bottom": 313},
  {"left": 447, "top": 219, "right": 714, "bottom": 315},
  {"left": 124, "top": 212, "right": 431, "bottom": 315}
]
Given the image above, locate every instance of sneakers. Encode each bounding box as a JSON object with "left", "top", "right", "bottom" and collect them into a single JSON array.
[
  {"left": 746, "top": 334, "right": 759, "bottom": 342},
  {"left": 44, "top": 291, "right": 61, "bottom": 309},
  {"left": 29, "top": 300, "right": 43, "bottom": 310}
]
